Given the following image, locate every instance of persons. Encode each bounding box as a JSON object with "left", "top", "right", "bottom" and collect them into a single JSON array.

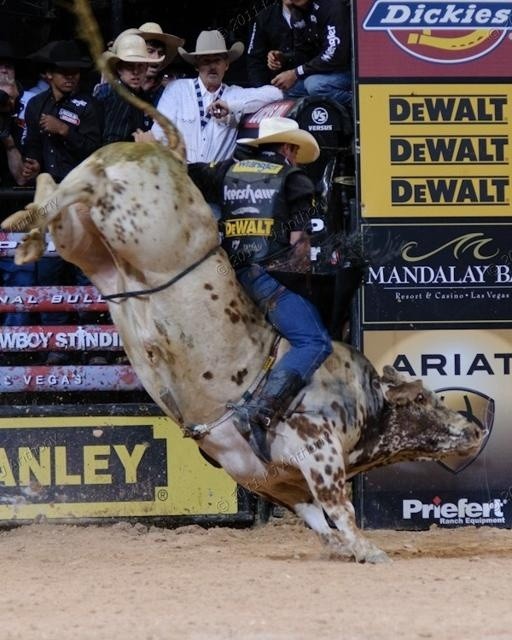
[
  {"left": 0, "top": 0, "right": 352, "bottom": 405},
  {"left": 185, "top": 141, "right": 333, "bottom": 465}
]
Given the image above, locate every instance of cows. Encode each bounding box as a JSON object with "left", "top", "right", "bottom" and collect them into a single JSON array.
[{"left": 0, "top": 0, "right": 486, "bottom": 567}]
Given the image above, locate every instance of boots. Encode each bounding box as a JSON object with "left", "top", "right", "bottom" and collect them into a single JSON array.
[{"left": 233, "top": 367, "right": 306, "bottom": 463}]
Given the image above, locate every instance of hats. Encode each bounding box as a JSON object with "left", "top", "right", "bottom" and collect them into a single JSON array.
[
  {"left": 178, "top": 30, "right": 244, "bottom": 64},
  {"left": 103, "top": 34, "right": 166, "bottom": 69},
  {"left": 27, "top": 40, "right": 91, "bottom": 73},
  {"left": 111, "top": 21, "right": 185, "bottom": 72},
  {"left": 236, "top": 117, "right": 320, "bottom": 164}
]
